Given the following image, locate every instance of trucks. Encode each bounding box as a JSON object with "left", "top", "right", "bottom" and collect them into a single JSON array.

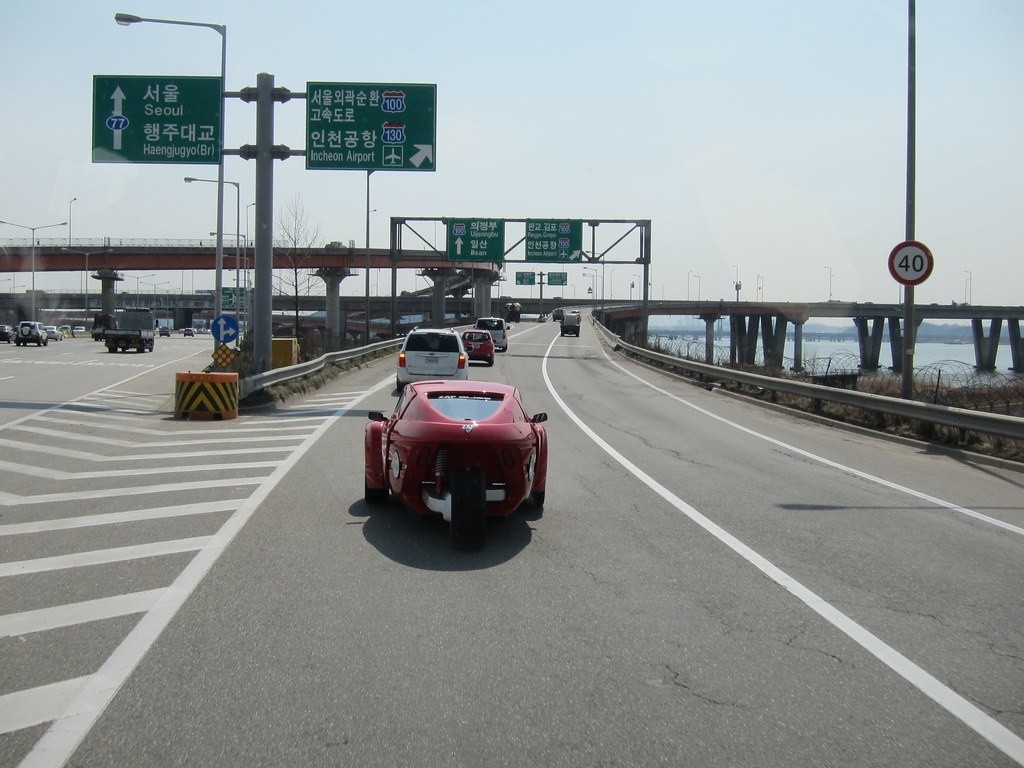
[
  {"left": 92, "top": 314, "right": 118, "bottom": 342},
  {"left": 560, "top": 314, "right": 581, "bottom": 338},
  {"left": 553, "top": 308, "right": 564, "bottom": 322},
  {"left": 103, "top": 307, "right": 155, "bottom": 353}
]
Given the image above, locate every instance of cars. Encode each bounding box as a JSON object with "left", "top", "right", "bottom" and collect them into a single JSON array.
[
  {"left": 0, "top": 326, "right": 86, "bottom": 343},
  {"left": 156, "top": 326, "right": 212, "bottom": 336},
  {"left": 571, "top": 310, "right": 580, "bottom": 314},
  {"left": 460, "top": 329, "right": 495, "bottom": 366},
  {"left": 474, "top": 316, "right": 511, "bottom": 352}
]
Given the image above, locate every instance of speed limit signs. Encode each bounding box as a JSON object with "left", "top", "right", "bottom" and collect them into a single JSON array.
[{"left": 888, "top": 241, "right": 935, "bottom": 286}]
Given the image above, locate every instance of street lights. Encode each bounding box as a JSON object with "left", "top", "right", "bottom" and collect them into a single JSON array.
[
  {"left": 366, "top": 210, "right": 377, "bottom": 248},
  {"left": 184, "top": 178, "right": 256, "bottom": 351},
  {"left": 497, "top": 251, "right": 973, "bottom": 308},
  {"left": 115, "top": 13, "right": 226, "bottom": 370},
  {"left": 0, "top": 197, "right": 181, "bottom": 327}
]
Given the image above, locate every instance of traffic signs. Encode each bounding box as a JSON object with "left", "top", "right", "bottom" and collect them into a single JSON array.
[
  {"left": 448, "top": 218, "right": 505, "bottom": 261},
  {"left": 306, "top": 81, "right": 437, "bottom": 172},
  {"left": 91, "top": 75, "right": 221, "bottom": 166},
  {"left": 526, "top": 218, "right": 582, "bottom": 263}
]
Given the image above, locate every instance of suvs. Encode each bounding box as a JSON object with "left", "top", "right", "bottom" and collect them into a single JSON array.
[
  {"left": 14, "top": 322, "right": 49, "bottom": 347},
  {"left": 396, "top": 326, "right": 469, "bottom": 394}
]
